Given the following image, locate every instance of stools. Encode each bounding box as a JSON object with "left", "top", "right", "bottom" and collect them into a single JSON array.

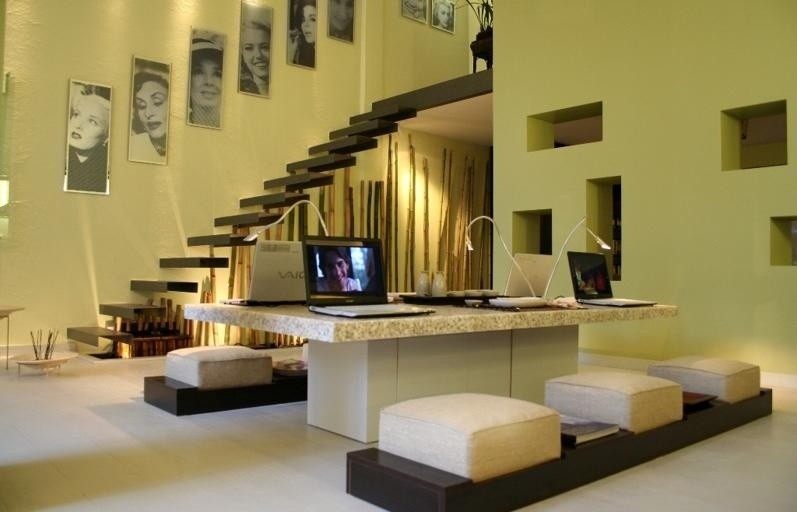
[
  {"left": 347, "top": 391, "right": 575, "bottom": 512},
  {"left": 544, "top": 370, "right": 685, "bottom": 470},
  {"left": 648, "top": 354, "right": 773, "bottom": 464},
  {"left": 143, "top": 345, "right": 273, "bottom": 417}
]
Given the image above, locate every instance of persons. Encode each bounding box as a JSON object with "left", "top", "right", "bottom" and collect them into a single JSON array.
[
  {"left": 434, "top": 0, "right": 452, "bottom": 29},
  {"left": 127, "top": 67, "right": 169, "bottom": 164},
  {"left": 315, "top": 246, "right": 360, "bottom": 291},
  {"left": 238, "top": 19, "right": 268, "bottom": 94},
  {"left": 67, "top": 92, "right": 107, "bottom": 190},
  {"left": 288, "top": 2, "right": 314, "bottom": 66},
  {"left": 190, "top": 34, "right": 222, "bottom": 128},
  {"left": 330, "top": 1, "right": 352, "bottom": 40}
]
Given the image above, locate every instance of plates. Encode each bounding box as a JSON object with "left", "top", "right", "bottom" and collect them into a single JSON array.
[
  {"left": 488, "top": 297, "right": 546, "bottom": 309},
  {"left": 272, "top": 361, "right": 307, "bottom": 377}
]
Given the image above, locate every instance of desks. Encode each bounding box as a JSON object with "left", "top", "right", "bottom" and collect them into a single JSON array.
[
  {"left": 183, "top": 294, "right": 678, "bottom": 444},
  {"left": 469, "top": 38, "right": 492, "bottom": 73},
  {"left": 0, "top": 302, "right": 25, "bottom": 378}
]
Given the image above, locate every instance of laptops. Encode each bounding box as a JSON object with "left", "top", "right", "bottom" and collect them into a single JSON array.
[
  {"left": 567, "top": 250, "right": 656, "bottom": 308},
  {"left": 497, "top": 253, "right": 554, "bottom": 297},
  {"left": 220, "top": 239, "right": 306, "bottom": 306},
  {"left": 301, "top": 232, "right": 436, "bottom": 318}
]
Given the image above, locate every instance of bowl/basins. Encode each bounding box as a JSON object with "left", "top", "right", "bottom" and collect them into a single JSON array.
[
  {"left": 464, "top": 300, "right": 482, "bottom": 307},
  {"left": 448, "top": 289, "right": 499, "bottom": 297}
]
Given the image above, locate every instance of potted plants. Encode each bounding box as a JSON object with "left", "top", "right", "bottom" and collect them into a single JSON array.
[{"left": 462, "top": 0, "right": 497, "bottom": 40}]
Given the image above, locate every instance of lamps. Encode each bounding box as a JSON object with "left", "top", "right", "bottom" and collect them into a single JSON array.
[
  {"left": 242, "top": 199, "right": 330, "bottom": 243},
  {"left": 464, "top": 214, "right": 612, "bottom": 307}
]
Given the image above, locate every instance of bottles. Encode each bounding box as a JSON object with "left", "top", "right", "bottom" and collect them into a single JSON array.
[
  {"left": 415, "top": 271, "right": 429, "bottom": 297},
  {"left": 432, "top": 271, "right": 447, "bottom": 297}
]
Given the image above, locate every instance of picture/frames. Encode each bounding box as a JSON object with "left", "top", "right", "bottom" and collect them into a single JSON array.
[
  {"left": 236, "top": 0, "right": 355, "bottom": 100},
  {"left": 431, "top": 0, "right": 456, "bottom": 34},
  {"left": 401, "top": 0, "right": 429, "bottom": 25},
  {"left": 62, "top": 23, "right": 227, "bottom": 196}
]
